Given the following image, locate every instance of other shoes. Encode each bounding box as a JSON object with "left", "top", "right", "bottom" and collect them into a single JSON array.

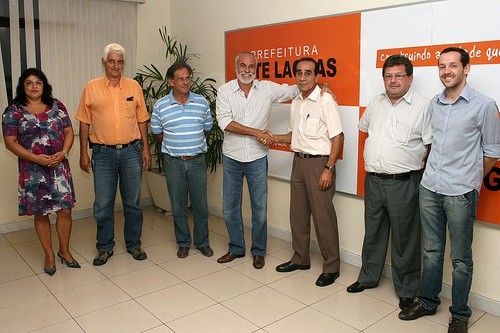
[
  {"left": 177, "top": 247, "right": 189, "bottom": 258},
  {"left": 196, "top": 245, "right": 213, "bottom": 257}
]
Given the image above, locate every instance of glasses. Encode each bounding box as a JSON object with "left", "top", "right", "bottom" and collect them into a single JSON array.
[{"left": 384, "top": 73, "right": 408, "bottom": 79}]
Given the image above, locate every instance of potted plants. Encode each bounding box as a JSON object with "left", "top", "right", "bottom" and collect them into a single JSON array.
[{"left": 131, "top": 28, "right": 222, "bottom": 215}]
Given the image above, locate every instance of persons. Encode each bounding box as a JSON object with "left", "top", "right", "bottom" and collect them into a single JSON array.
[
  {"left": 1, "top": 67, "right": 81, "bottom": 276},
  {"left": 216, "top": 52, "right": 337, "bottom": 270},
  {"left": 74, "top": 43, "right": 152, "bottom": 265},
  {"left": 256, "top": 58, "right": 346, "bottom": 287},
  {"left": 399, "top": 47, "right": 499, "bottom": 333},
  {"left": 347, "top": 55, "right": 432, "bottom": 312},
  {"left": 151, "top": 62, "right": 214, "bottom": 259}
]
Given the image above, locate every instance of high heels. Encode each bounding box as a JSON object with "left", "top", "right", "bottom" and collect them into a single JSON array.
[
  {"left": 57, "top": 251, "right": 81, "bottom": 268},
  {"left": 44, "top": 254, "right": 56, "bottom": 276}
]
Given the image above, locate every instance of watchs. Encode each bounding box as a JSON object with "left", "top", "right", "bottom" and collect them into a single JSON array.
[
  {"left": 324, "top": 165, "right": 334, "bottom": 172},
  {"left": 62, "top": 151, "right": 68, "bottom": 159}
]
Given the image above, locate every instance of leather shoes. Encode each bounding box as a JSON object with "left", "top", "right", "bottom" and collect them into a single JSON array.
[
  {"left": 253, "top": 255, "right": 265, "bottom": 269},
  {"left": 399, "top": 297, "right": 414, "bottom": 310},
  {"left": 275, "top": 261, "right": 310, "bottom": 272},
  {"left": 347, "top": 281, "right": 378, "bottom": 293},
  {"left": 316, "top": 271, "right": 340, "bottom": 287},
  {"left": 217, "top": 252, "right": 245, "bottom": 263}
]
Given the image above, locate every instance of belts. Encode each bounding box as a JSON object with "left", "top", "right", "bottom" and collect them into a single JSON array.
[
  {"left": 295, "top": 152, "right": 329, "bottom": 158},
  {"left": 95, "top": 139, "right": 138, "bottom": 150},
  {"left": 177, "top": 153, "right": 203, "bottom": 160},
  {"left": 368, "top": 172, "right": 410, "bottom": 180}
]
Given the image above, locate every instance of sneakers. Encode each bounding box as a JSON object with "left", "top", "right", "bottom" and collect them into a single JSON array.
[
  {"left": 447, "top": 317, "right": 468, "bottom": 333},
  {"left": 93, "top": 250, "right": 113, "bottom": 266},
  {"left": 398, "top": 301, "right": 436, "bottom": 321},
  {"left": 127, "top": 247, "right": 147, "bottom": 260}
]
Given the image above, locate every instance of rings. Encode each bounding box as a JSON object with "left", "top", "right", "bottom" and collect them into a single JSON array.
[{"left": 327, "top": 184, "right": 329, "bottom": 186}]
